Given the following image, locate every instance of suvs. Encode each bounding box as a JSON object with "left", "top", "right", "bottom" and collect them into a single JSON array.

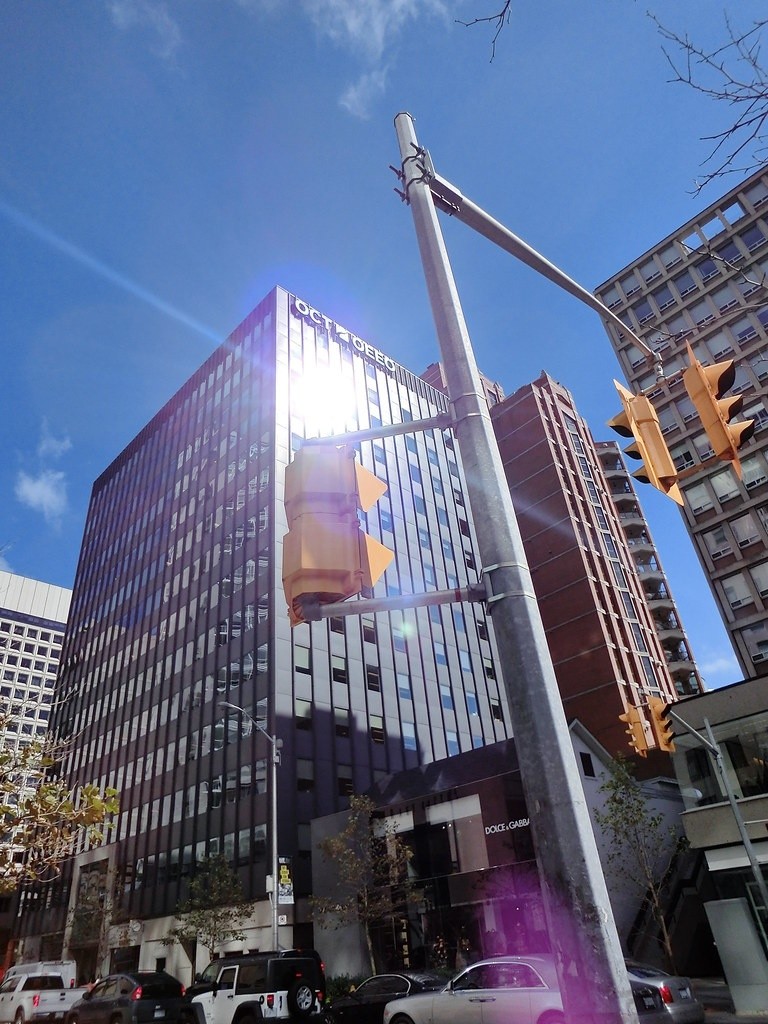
[{"left": 185, "top": 949, "right": 325, "bottom": 1024}]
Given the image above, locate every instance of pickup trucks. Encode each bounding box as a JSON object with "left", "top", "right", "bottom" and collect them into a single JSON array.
[{"left": 0, "top": 972, "right": 88, "bottom": 1024}]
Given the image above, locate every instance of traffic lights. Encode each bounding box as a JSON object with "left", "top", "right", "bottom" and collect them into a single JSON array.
[
  {"left": 683, "top": 340, "right": 755, "bottom": 480},
  {"left": 606, "top": 379, "right": 685, "bottom": 508},
  {"left": 619, "top": 703, "right": 648, "bottom": 758},
  {"left": 646, "top": 696, "right": 676, "bottom": 752}
]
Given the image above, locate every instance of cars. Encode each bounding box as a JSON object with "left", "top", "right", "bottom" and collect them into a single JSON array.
[
  {"left": 66, "top": 972, "right": 186, "bottom": 1024},
  {"left": 330, "top": 973, "right": 446, "bottom": 1024},
  {"left": 384, "top": 955, "right": 705, "bottom": 1024}
]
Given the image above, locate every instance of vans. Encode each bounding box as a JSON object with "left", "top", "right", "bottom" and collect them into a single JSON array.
[{"left": 0, "top": 960, "right": 76, "bottom": 990}]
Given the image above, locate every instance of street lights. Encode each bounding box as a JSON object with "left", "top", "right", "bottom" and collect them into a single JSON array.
[{"left": 216, "top": 702, "right": 283, "bottom": 951}]
